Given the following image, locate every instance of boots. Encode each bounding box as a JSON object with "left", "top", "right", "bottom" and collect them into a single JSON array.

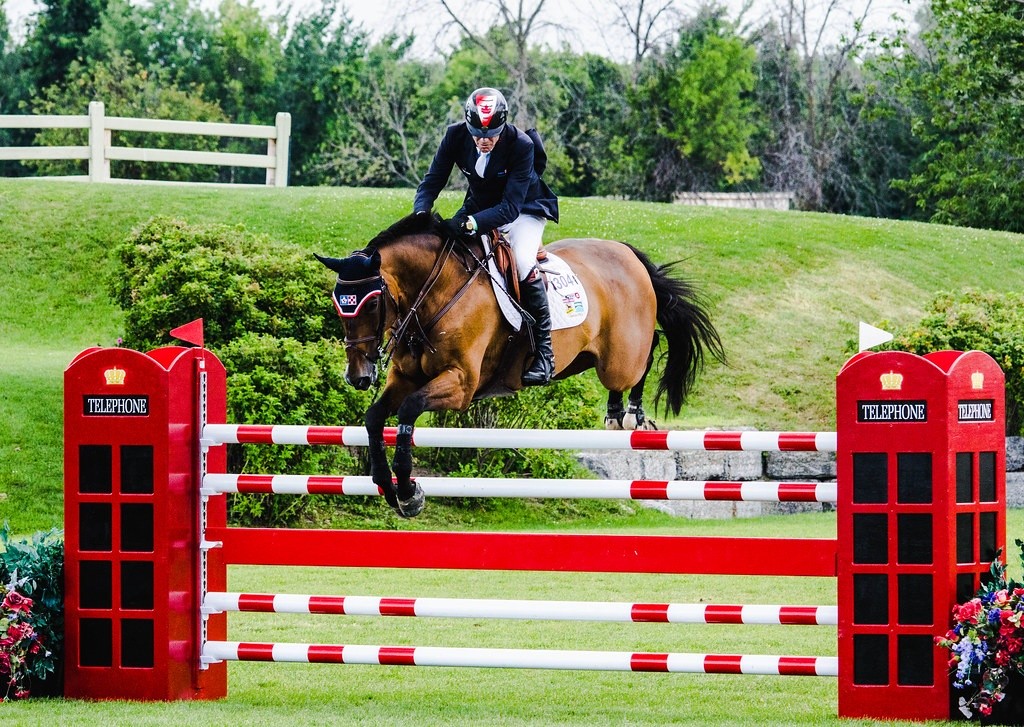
[{"left": 518, "top": 263, "right": 555, "bottom": 385}]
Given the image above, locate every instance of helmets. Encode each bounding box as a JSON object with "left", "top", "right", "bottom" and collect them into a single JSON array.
[{"left": 464, "top": 87, "right": 508, "bottom": 136}]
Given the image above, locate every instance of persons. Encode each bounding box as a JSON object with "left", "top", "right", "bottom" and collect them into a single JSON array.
[{"left": 412, "top": 86, "right": 564, "bottom": 385}]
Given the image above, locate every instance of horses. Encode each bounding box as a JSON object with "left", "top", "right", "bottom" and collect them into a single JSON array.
[{"left": 314, "top": 210, "right": 731, "bottom": 518}]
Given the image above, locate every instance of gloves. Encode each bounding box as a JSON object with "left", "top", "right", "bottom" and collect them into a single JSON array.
[{"left": 440, "top": 214, "right": 473, "bottom": 234}]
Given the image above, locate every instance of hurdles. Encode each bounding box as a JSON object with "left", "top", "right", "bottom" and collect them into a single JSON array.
[{"left": 199, "top": 416, "right": 841, "bottom": 683}]
